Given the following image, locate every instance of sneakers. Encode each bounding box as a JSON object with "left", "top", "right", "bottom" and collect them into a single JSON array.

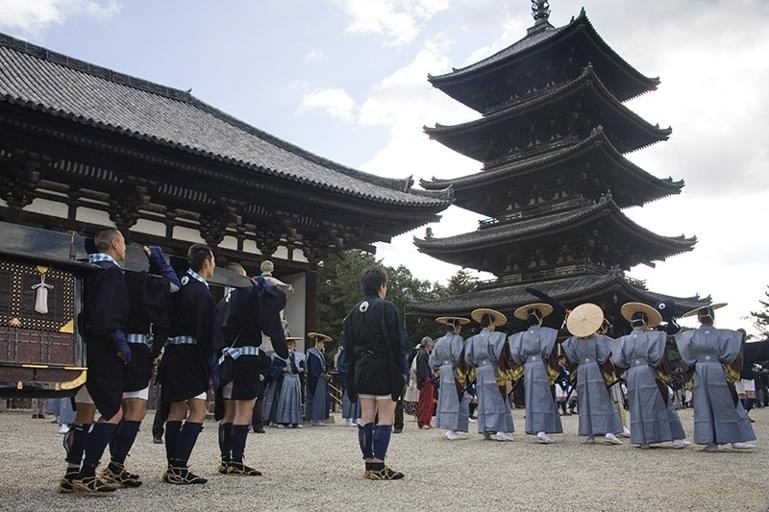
[
  {"left": 32, "top": 413, "right": 45, "bottom": 418},
  {"left": 163, "top": 460, "right": 262, "bottom": 483},
  {"left": 344, "top": 421, "right": 358, "bottom": 427},
  {"left": 253, "top": 422, "right": 325, "bottom": 433},
  {"left": 363, "top": 461, "right": 404, "bottom": 479},
  {"left": 57, "top": 461, "right": 142, "bottom": 496}
]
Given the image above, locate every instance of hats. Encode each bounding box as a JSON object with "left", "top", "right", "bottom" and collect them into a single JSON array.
[
  {"left": 566, "top": 302, "right": 662, "bottom": 337},
  {"left": 435, "top": 302, "right": 553, "bottom": 327},
  {"left": 682, "top": 302, "right": 728, "bottom": 317},
  {"left": 285, "top": 332, "right": 333, "bottom": 343}
]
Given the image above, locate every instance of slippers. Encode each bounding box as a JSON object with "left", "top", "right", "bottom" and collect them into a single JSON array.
[
  {"left": 445, "top": 432, "right": 553, "bottom": 443},
  {"left": 580, "top": 425, "right": 757, "bottom": 452}
]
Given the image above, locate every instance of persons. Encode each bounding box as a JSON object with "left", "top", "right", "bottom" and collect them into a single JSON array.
[
  {"left": 403, "top": 302, "right": 769, "bottom": 451},
  {"left": 343, "top": 267, "right": 410, "bottom": 482},
  {"left": 272, "top": 333, "right": 362, "bottom": 429},
  {"left": 33, "top": 225, "right": 292, "bottom": 494}
]
